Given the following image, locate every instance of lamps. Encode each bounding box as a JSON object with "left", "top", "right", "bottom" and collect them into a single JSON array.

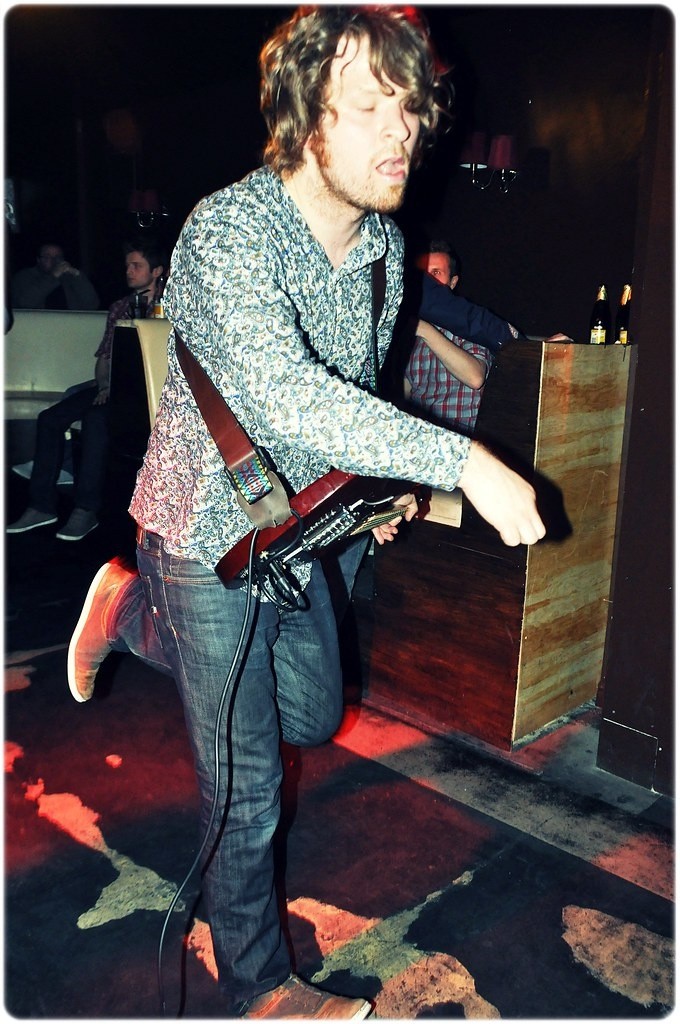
[
  {"left": 459, "top": 131, "right": 520, "bottom": 193},
  {"left": 128, "top": 190, "right": 169, "bottom": 228}
]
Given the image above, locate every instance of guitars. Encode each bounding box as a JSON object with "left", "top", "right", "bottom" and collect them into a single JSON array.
[{"left": 215, "top": 462, "right": 432, "bottom": 588}]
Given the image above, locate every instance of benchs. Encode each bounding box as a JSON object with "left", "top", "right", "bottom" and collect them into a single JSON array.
[
  {"left": 340, "top": 368, "right": 632, "bottom": 752},
  {"left": 3, "top": 308, "right": 109, "bottom": 486},
  {"left": 106, "top": 318, "right": 173, "bottom": 556}
]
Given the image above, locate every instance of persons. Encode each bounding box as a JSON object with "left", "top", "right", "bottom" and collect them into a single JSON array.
[
  {"left": 11, "top": 241, "right": 100, "bottom": 310},
  {"left": 6, "top": 232, "right": 172, "bottom": 541},
  {"left": 327, "top": 240, "right": 493, "bottom": 604},
  {"left": 67, "top": 5, "right": 546, "bottom": 1019}
]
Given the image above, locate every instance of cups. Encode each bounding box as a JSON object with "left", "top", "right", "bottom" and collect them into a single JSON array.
[
  {"left": 129, "top": 295, "right": 148, "bottom": 319},
  {"left": 52, "top": 254, "right": 69, "bottom": 270}
]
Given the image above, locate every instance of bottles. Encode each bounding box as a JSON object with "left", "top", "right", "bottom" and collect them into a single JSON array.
[
  {"left": 589, "top": 284, "right": 610, "bottom": 344},
  {"left": 153, "top": 275, "right": 167, "bottom": 319},
  {"left": 615, "top": 283, "right": 632, "bottom": 345}
]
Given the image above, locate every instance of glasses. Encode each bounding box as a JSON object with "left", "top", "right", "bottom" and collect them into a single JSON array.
[{"left": 38, "top": 254, "right": 61, "bottom": 263}]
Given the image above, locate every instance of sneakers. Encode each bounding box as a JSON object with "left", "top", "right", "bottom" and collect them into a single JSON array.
[
  {"left": 68, "top": 562, "right": 133, "bottom": 703},
  {"left": 6, "top": 508, "right": 58, "bottom": 533},
  {"left": 56, "top": 509, "right": 99, "bottom": 541},
  {"left": 238, "top": 974, "right": 372, "bottom": 1021}
]
